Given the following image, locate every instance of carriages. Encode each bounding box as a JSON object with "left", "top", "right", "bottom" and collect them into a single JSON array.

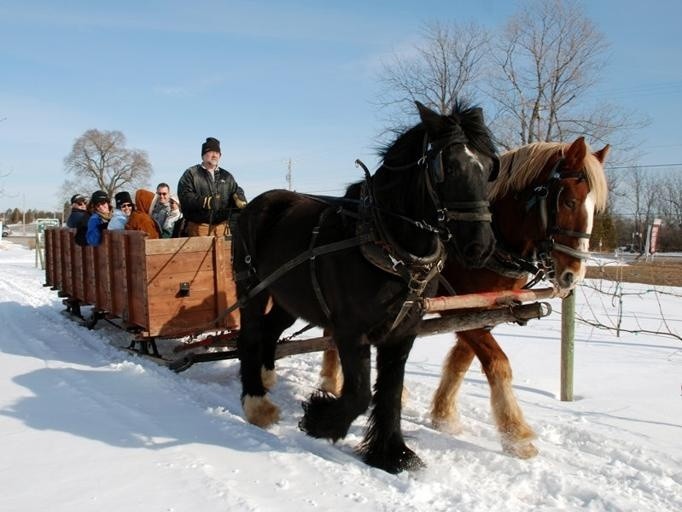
[{"left": 43, "top": 101, "right": 611, "bottom": 475}]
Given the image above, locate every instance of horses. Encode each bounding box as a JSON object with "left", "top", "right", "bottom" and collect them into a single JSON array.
[
  {"left": 231, "top": 97, "right": 497, "bottom": 475},
  {"left": 320, "top": 135, "right": 613, "bottom": 460}
]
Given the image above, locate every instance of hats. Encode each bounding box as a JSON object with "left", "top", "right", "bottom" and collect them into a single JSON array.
[
  {"left": 93, "top": 191, "right": 108, "bottom": 203},
  {"left": 115, "top": 192, "right": 132, "bottom": 207},
  {"left": 169, "top": 192, "right": 180, "bottom": 204},
  {"left": 201, "top": 137, "right": 221, "bottom": 156},
  {"left": 71, "top": 194, "right": 88, "bottom": 202}
]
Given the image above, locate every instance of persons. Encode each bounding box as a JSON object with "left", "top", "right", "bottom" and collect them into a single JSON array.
[
  {"left": 66, "top": 182, "right": 181, "bottom": 247},
  {"left": 179, "top": 138, "right": 246, "bottom": 236}
]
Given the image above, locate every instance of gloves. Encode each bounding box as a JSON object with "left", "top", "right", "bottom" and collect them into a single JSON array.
[
  {"left": 199, "top": 194, "right": 221, "bottom": 211},
  {"left": 233, "top": 193, "right": 249, "bottom": 210}
]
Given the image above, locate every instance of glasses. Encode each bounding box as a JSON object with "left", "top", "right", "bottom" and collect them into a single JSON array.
[
  {"left": 168, "top": 201, "right": 176, "bottom": 204},
  {"left": 156, "top": 192, "right": 167, "bottom": 196},
  {"left": 77, "top": 201, "right": 87, "bottom": 206},
  {"left": 121, "top": 203, "right": 132, "bottom": 208}
]
